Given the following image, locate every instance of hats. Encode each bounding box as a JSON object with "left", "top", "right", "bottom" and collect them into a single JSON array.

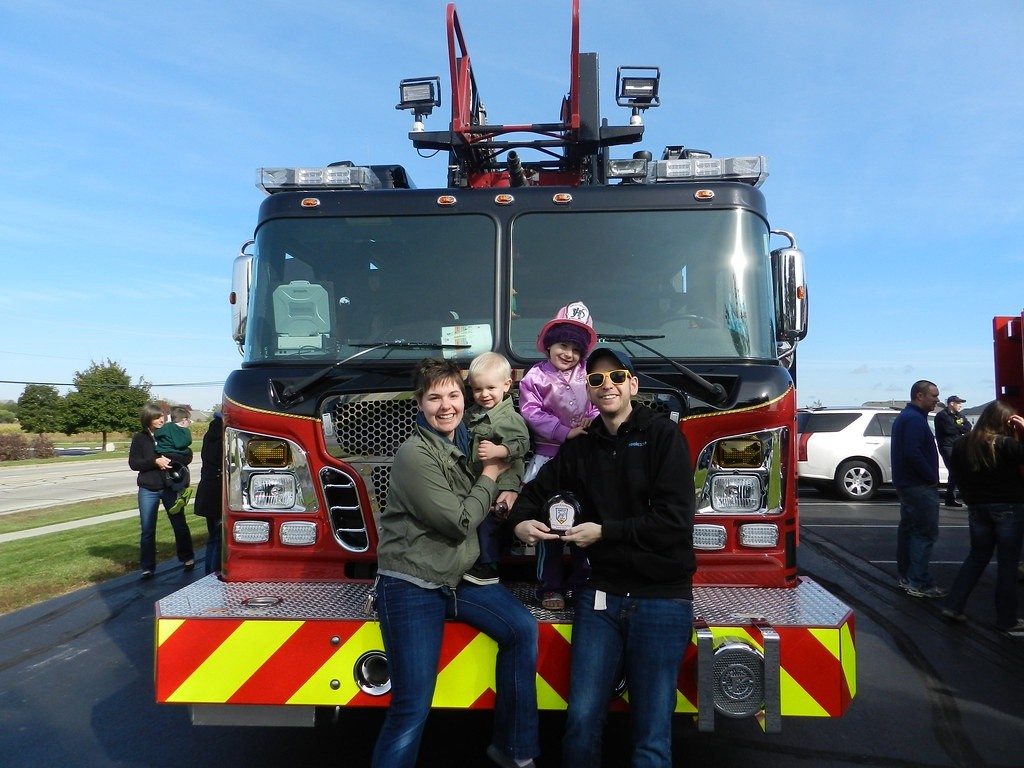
[
  {"left": 585, "top": 347, "right": 635, "bottom": 377},
  {"left": 167, "top": 461, "right": 190, "bottom": 491},
  {"left": 543, "top": 322, "right": 591, "bottom": 356},
  {"left": 947, "top": 396, "right": 966, "bottom": 403},
  {"left": 536, "top": 301, "right": 597, "bottom": 355}
]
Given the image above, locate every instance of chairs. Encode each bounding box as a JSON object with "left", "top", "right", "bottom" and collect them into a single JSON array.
[{"left": 336, "top": 251, "right": 427, "bottom": 340}]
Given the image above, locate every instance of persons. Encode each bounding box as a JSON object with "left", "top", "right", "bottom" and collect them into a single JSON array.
[
  {"left": 153, "top": 407, "right": 192, "bottom": 514},
  {"left": 373, "top": 357, "right": 542, "bottom": 768},
  {"left": 194, "top": 408, "right": 225, "bottom": 577},
  {"left": 890, "top": 380, "right": 951, "bottom": 598},
  {"left": 940, "top": 397, "right": 1024, "bottom": 638},
  {"left": 464, "top": 352, "right": 530, "bottom": 585},
  {"left": 509, "top": 347, "right": 698, "bottom": 768},
  {"left": 518, "top": 302, "right": 599, "bottom": 610},
  {"left": 129, "top": 406, "right": 196, "bottom": 581},
  {"left": 934, "top": 395, "right": 974, "bottom": 508}
]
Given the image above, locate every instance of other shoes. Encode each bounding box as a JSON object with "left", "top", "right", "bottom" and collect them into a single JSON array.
[
  {"left": 945, "top": 499, "right": 962, "bottom": 507},
  {"left": 169, "top": 499, "right": 185, "bottom": 515},
  {"left": 141, "top": 570, "right": 154, "bottom": 580},
  {"left": 995, "top": 618, "right": 1024, "bottom": 637},
  {"left": 942, "top": 605, "right": 967, "bottom": 621},
  {"left": 487, "top": 744, "right": 536, "bottom": 768},
  {"left": 182, "top": 487, "right": 193, "bottom": 504},
  {"left": 185, "top": 558, "right": 194, "bottom": 571}
]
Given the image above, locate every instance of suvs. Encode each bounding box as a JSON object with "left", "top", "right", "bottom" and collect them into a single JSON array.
[{"left": 796, "top": 406, "right": 950, "bottom": 503}]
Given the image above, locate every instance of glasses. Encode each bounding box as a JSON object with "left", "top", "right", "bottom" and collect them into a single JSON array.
[{"left": 587, "top": 369, "right": 632, "bottom": 388}]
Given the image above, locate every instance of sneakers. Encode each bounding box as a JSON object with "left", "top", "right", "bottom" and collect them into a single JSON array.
[
  {"left": 907, "top": 585, "right": 949, "bottom": 598},
  {"left": 897, "top": 576, "right": 912, "bottom": 590},
  {"left": 463, "top": 564, "right": 499, "bottom": 586}
]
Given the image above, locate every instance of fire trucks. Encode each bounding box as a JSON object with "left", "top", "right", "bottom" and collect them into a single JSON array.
[{"left": 152, "top": 1, "right": 860, "bottom": 728}]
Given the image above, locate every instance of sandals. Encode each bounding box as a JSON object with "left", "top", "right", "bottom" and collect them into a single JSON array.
[{"left": 542, "top": 591, "right": 565, "bottom": 610}]
